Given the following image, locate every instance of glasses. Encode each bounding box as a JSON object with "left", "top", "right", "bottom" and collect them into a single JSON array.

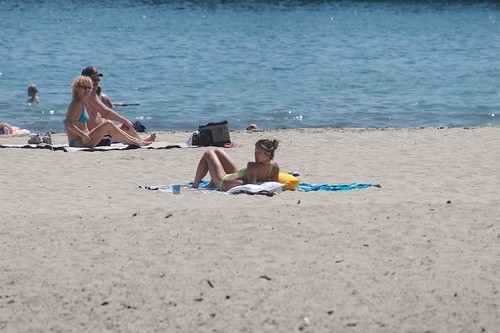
[
  {"left": 80, "top": 86, "right": 93, "bottom": 90},
  {"left": 92, "top": 77, "right": 100, "bottom": 81}
]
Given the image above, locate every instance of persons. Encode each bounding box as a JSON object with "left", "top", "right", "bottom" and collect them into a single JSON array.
[
  {"left": 95, "top": 86, "right": 114, "bottom": 108},
  {"left": 191, "top": 139, "right": 279, "bottom": 192},
  {"left": 82, "top": 67, "right": 156, "bottom": 143},
  {"left": 0, "top": 123, "right": 20, "bottom": 134},
  {"left": 27, "top": 85, "right": 40, "bottom": 103},
  {"left": 64, "top": 75, "right": 152, "bottom": 148}
]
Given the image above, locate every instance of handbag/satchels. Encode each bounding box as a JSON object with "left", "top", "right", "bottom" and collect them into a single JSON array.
[{"left": 198, "top": 119, "right": 231, "bottom": 146}]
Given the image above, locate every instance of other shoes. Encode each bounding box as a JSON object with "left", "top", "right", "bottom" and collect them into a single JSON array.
[
  {"left": 42, "top": 132, "right": 52, "bottom": 143},
  {"left": 28, "top": 133, "right": 43, "bottom": 144}
]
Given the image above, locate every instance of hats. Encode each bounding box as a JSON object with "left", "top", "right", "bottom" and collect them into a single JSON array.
[{"left": 82, "top": 67, "right": 103, "bottom": 77}]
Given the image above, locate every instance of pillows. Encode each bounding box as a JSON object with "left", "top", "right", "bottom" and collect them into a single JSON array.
[{"left": 278, "top": 172, "right": 300, "bottom": 190}]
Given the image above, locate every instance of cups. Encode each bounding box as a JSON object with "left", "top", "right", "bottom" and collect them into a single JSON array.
[{"left": 171, "top": 184, "right": 181, "bottom": 195}]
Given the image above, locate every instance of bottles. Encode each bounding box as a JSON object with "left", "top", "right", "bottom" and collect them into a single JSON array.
[{"left": 192, "top": 131, "right": 210, "bottom": 145}]
